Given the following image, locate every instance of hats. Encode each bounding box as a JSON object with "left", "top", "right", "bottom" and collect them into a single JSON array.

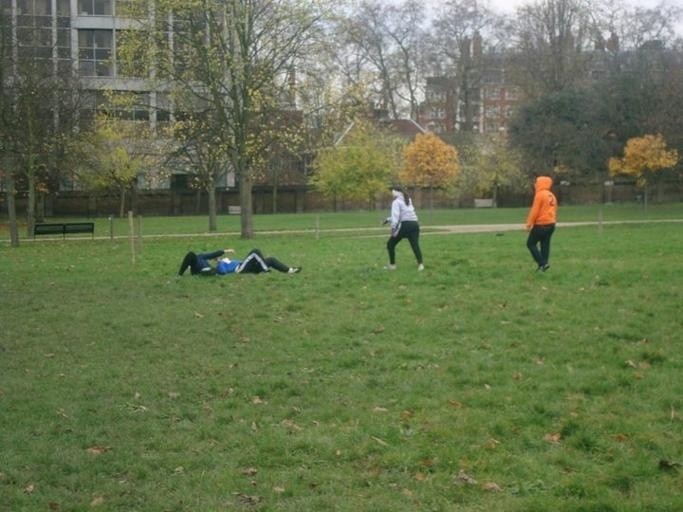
[{"left": 386, "top": 184, "right": 400, "bottom": 191}]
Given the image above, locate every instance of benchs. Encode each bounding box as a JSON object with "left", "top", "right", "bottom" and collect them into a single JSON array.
[{"left": 34, "top": 222, "right": 94, "bottom": 241}]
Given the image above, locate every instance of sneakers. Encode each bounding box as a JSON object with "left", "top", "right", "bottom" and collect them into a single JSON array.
[
  {"left": 416, "top": 264, "right": 425, "bottom": 272},
  {"left": 535, "top": 263, "right": 550, "bottom": 273},
  {"left": 286, "top": 266, "right": 301, "bottom": 274},
  {"left": 382, "top": 264, "right": 397, "bottom": 271}
]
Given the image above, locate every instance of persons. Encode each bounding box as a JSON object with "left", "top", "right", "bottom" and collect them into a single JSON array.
[
  {"left": 382, "top": 184, "right": 424, "bottom": 271},
  {"left": 174, "top": 249, "right": 234, "bottom": 282},
  {"left": 526, "top": 175, "right": 557, "bottom": 270},
  {"left": 216, "top": 248, "right": 302, "bottom": 273}
]
